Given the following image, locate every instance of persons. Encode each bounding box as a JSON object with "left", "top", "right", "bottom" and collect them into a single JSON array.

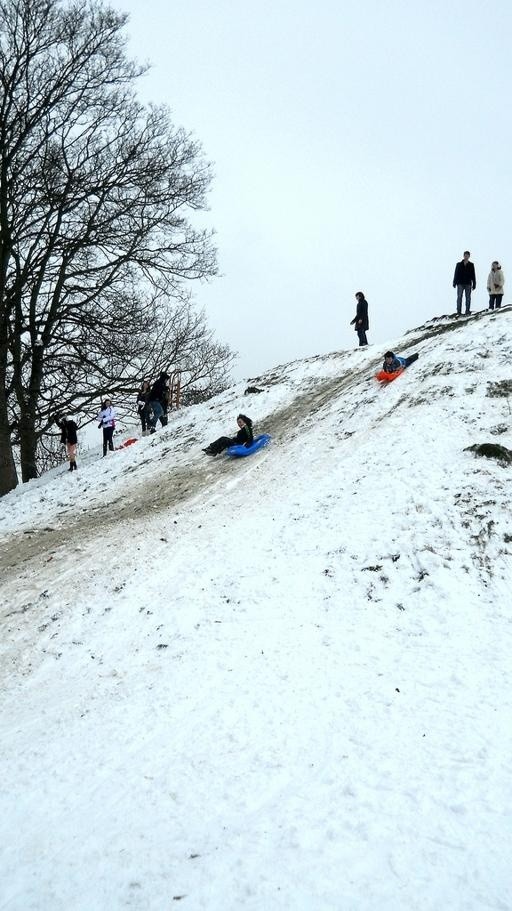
[
  {"left": 54, "top": 412, "right": 79, "bottom": 471},
  {"left": 97, "top": 400, "right": 115, "bottom": 458},
  {"left": 137, "top": 372, "right": 170, "bottom": 437},
  {"left": 453, "top": 251, "right": 476, "bottom": 315},
  {"left": 383, "top": 352, "right": 419, "bottom": 375},
  {"left": 201, "top": 414, "right": 253, "bottom": 458},
  {"left": 351, "top": 292, "right": 369, "bottom": 346},
  {"left": 486, "top": 261, "right": 504, "bottom": 309}
]
